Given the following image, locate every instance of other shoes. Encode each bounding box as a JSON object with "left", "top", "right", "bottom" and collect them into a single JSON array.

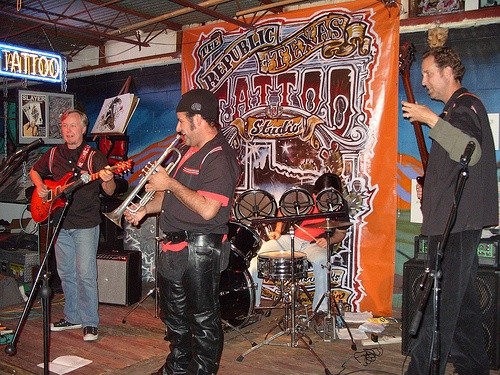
[{"left": 313, "top": 310, "right": 328, "bottom": 326}]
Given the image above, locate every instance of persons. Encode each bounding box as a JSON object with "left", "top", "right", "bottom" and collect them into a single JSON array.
[
  {"left": 30, "top": 110, "right": 116, "bottom": 341},
  {"left": 248, "top": 173, "right": 351, "bottom": 332},
  {"left": 123, "top": 88, "right": 239, "bottom": 375},
  {"left": 401, "top": 45, "right": 499, "bottom": 375}
]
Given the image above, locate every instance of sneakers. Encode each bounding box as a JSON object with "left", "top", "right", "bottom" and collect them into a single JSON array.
[
  {"left": 83, "top": 326, "right": 99, "bottom": 340},
  {"left": 50, "top": 319, "right": 82, "bottom": 331}
]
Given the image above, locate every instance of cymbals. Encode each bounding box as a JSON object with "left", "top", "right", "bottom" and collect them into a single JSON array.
[{"left": 303, "top": 220, "right": 355, "bottom": 230}]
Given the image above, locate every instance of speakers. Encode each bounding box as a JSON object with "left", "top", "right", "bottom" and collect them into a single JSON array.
[
  {"left": 50, "top": 202, "right": 123, "bottom": 294},
  {"left": 96, "top": 250, "right": 142, "bottom": 307},
  {"left": 401, "top": 258, "right": 500, "bottom": 371}
]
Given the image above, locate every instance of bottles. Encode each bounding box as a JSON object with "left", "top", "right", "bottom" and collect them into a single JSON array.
[
  {"left": 336, "top": 300, "right": 345, "bottom": 329},
  {"left": 324, "top": 312, "right": 336, "bottom": 342}
]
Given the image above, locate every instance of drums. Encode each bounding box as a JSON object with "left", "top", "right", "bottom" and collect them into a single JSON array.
[
  {"left": 227, "top": 220, "right": 262, "bottom": 270},
  {"left": 234, "top": 189, "right": 278, "bottom": 227},
  {"left": 257, "top": 251, "right": 308, "bottom": 280},
  {"left": 316, "top": 188, "right": 343, "bottom": 214},
  {"left": 218, "top": 267, "right": 256, "bottom": 331},
  {"left": 280, "top": 188, "right": 315, "bottom": 216}
]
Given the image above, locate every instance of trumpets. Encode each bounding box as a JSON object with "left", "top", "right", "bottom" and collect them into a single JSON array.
[{"left": 102, "top": 133, "right": 184, "bottom": 228}]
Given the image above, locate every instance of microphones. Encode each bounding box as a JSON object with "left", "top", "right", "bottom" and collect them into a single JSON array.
[
  {"left": 13, "top": 138, "right": 44, "bottom": 155},
  {"left": 458, "top": 141, "right": 476, "bottom": 167},
  {"left": 54, "top": 172, "right": 91, "bottom": 198}
]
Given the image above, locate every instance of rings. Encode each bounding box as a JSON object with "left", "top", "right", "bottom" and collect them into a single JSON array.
[{"left": 406, "top": 113, "right": 409, "bottom": 118}]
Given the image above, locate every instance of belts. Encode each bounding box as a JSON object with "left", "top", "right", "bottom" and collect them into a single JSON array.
[{"left": 162, "top": 231, "right": 224, "bottom": 247}]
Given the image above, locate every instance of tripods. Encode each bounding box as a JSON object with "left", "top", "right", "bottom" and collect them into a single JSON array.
[{"left": 219, "top": 220, "right": 357, "bottom": 375}]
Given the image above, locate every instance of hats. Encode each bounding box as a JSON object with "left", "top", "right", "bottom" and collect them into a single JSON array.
[{"left": 176, "top": 89, "right": 219, "bottom": 119}]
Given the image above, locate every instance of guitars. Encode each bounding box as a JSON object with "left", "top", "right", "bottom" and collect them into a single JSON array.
[
  {"left": 398, "top": 42, "right": 429, "bottom": 209},
  {"left": 30, "top": 160, "right": 133, "bottom": 223}
]
